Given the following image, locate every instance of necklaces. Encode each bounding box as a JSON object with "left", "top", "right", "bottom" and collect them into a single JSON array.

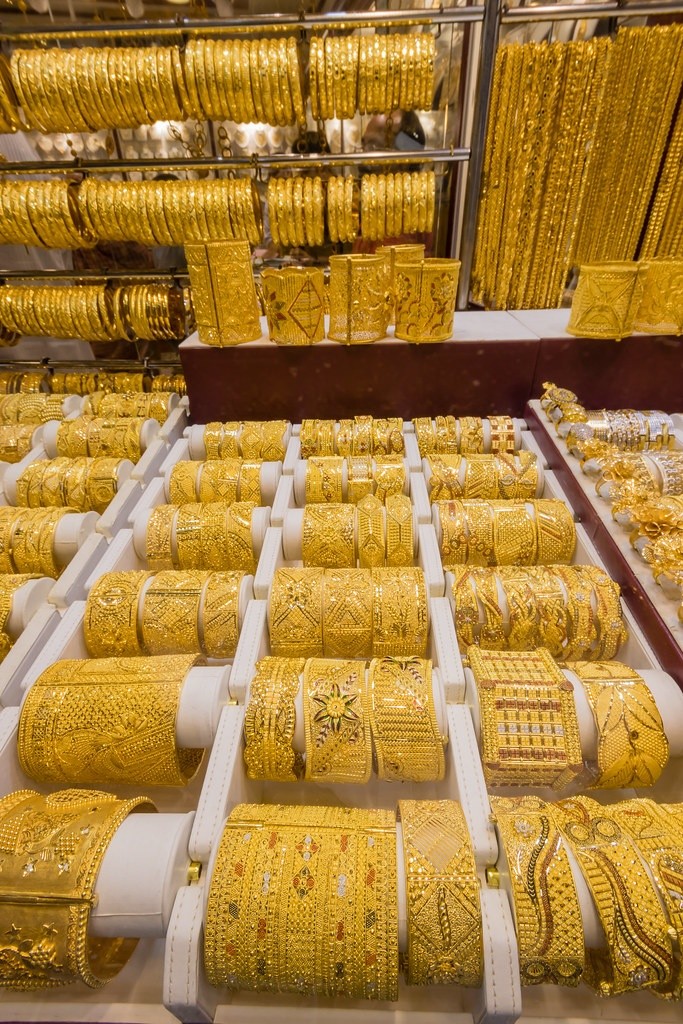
[{"left": 471, "top": 24, "right": 683, "bottom": 312}]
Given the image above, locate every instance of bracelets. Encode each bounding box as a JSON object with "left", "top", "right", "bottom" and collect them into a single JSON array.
[
  {"left": 0, "top": 414, "right": 683, "bottom": 1024},
  {"left": 268, "top": 171, "right": 436, "bottom": 247},
  {"left": 0, "top": 284, "right": 197, "bottom": 342},
  {"left": 0, "top": 34, "right": 437, "bottom": 134},
  {"left": 0, "top": 368, "right": 186, "bottom": 667},
  {"left": 184, "top": 240, "right": 461, "bottom": 348},
  {"left": 587, "top": 408, "right": 683, "bottom": 497},
  {"left": 565, "top": 256, "right": 683, "bottom": 338},
  {"left": 0, "top": 178, "right": 263, "bottom": 249}
]
